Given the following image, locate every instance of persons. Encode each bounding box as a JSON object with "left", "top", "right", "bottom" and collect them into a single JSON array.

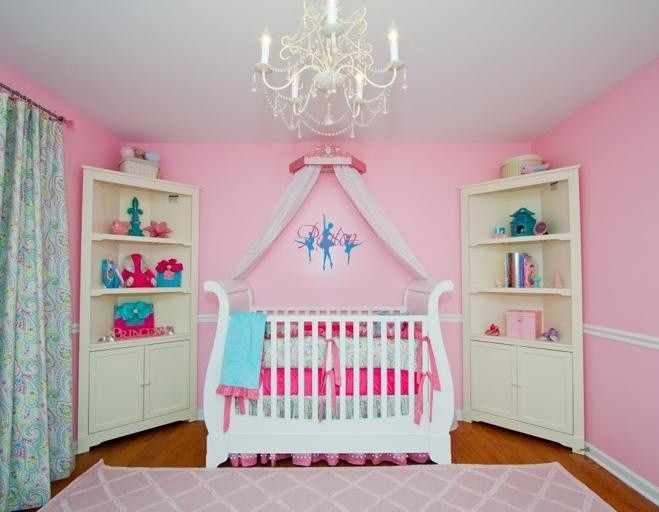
[{"left": 297, "top": 213, "right": 365, "bottom": 274}]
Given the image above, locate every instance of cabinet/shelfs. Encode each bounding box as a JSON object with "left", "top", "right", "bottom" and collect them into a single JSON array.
[
  {"left": 76, "top": 164, "right": 203, "bottom": 455},
  {"left": 457, "top": 165, "right": 585, "bottom": 455}
]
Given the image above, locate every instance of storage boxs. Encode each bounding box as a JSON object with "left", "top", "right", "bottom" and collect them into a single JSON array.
[{"left": 505, "top": 309, "right": 542, "bottom": 339}]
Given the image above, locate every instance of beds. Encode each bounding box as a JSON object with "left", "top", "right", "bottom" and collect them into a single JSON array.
[{"left": 202, "top": 276, "right": 456, "bottom": 469}]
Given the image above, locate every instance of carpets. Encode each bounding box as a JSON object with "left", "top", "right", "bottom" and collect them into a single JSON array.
[{"left": 35, "top": 460, "right": 621, "bottom": 512}]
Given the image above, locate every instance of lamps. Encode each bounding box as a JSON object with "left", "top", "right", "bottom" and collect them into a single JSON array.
[{"left": 249, "top": 1, "right": 409, "bottom": 141}]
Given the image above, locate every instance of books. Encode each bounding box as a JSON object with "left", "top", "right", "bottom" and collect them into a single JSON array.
[{"left": 505, "top": 252, "right": 528, "bottom": 289}]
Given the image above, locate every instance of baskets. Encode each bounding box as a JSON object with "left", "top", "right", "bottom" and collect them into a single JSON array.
[
  {"left": 500, "top": 155, "right": 544, "bottom": 178},
  {"left": 119, "top": 158, "right": 160, "bottom": 179}
]
